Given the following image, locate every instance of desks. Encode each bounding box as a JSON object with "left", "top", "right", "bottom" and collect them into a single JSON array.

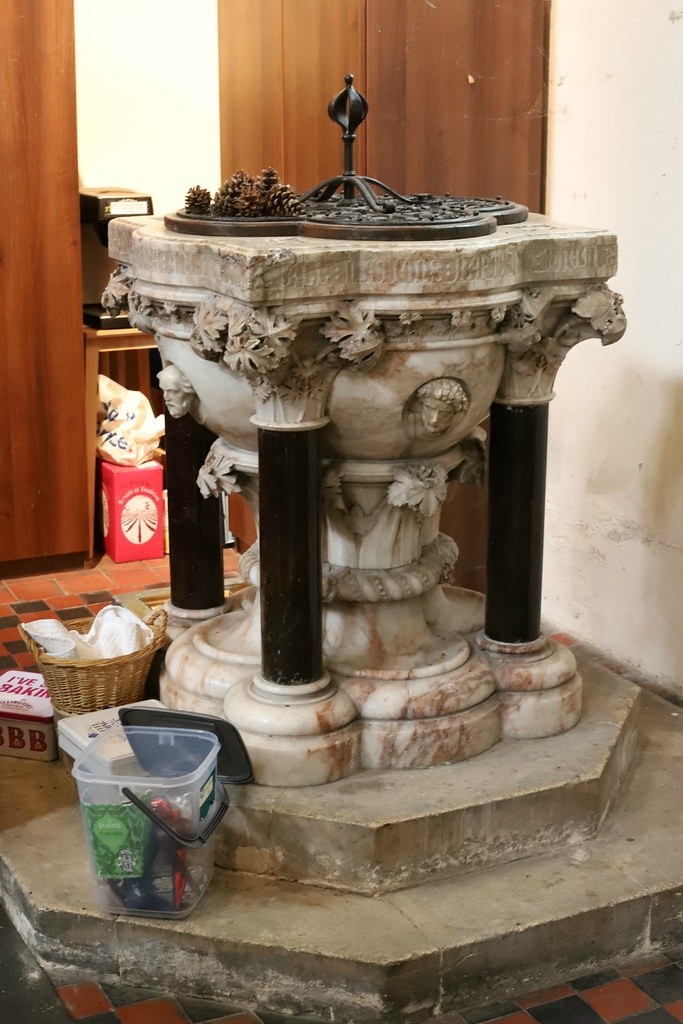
[{"left": 86, "top": 324, "right": 163, "bottom": 570}]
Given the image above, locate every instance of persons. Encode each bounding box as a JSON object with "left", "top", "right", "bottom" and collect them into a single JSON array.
[
  {"left": 156, "top": 365, "right": 195, "bottom": 419},
  {"left": 406, "top": 379, "right": 469, "bottom": 441}
]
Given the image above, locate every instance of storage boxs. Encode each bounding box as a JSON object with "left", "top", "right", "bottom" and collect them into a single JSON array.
[
  {"left": 101, "top": 456, "right": 162, "bottom": 567},
  {"left": 0, "top": 671, "right": 59, "bottom": 762},
  {"left": 58, "top": 697, "right": 167, "bottom": 782}
]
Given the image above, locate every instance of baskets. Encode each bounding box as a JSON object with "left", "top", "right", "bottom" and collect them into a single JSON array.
[{"left": 17, "top": 608, "right": 168, "bottom": 718}]
